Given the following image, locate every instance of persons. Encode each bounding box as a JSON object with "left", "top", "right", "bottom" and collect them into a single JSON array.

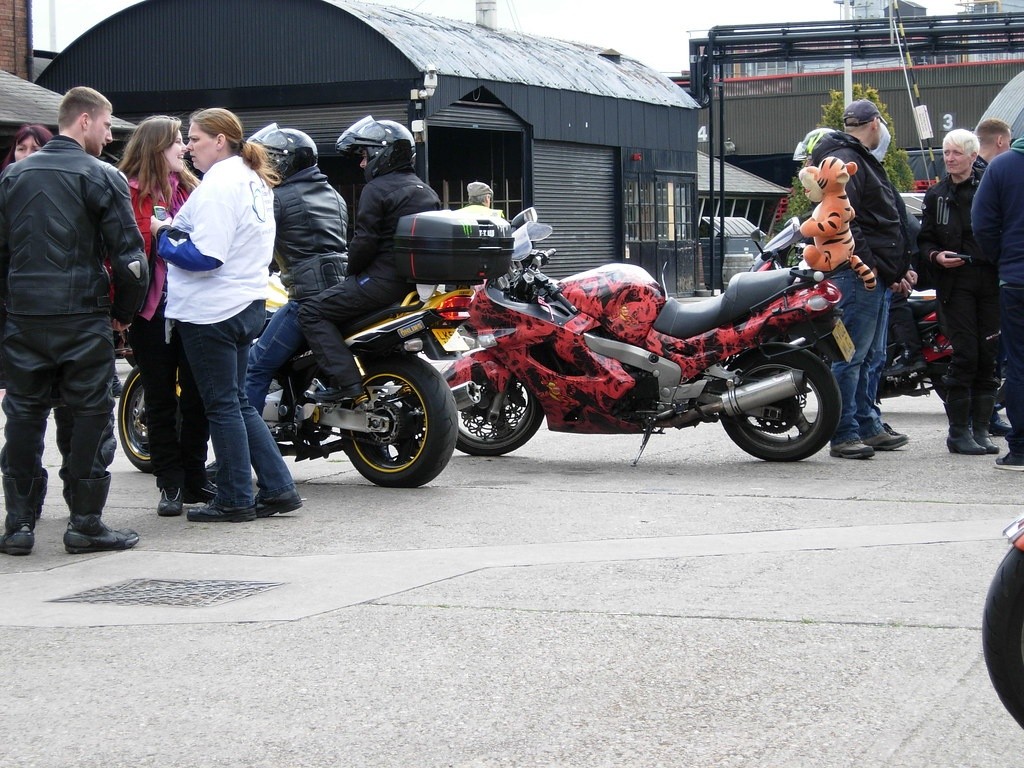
[
  {"left": 205, "top": 115, "right": 443, "bottom": 484},
  {"left": 112, "top": 107, "right": 304, "bottom": 522},
  {"left": 451, "top": 182, "right": 506, "bottom": 219},
  {"left": 921, "top": 118, "right": 1024, "bottom": 473},
  {"left": 0, "top": 85, "right": 150, "bottom": 555},
  {"left": 813, "top": 101, "right": 918, "bottom": 460}
]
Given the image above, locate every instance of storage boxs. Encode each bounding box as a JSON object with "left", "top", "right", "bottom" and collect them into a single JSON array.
[{"left": 394, "top": 210, "right": 515, "bottom": 282}]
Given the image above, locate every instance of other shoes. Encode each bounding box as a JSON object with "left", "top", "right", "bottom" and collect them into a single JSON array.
[{"left": 114, "top": 385, "right": 124, "bottom": 397}]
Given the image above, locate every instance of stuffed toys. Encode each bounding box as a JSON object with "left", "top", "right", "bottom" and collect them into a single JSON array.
[{"left": 799, "top": 156, "right": 877, "bottom": 291}]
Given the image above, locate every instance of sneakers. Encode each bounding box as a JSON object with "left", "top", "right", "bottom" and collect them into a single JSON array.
[
  {"left": 315, "top": 383, "right": 363, "bottom": 400},
  {"left": 255, "top": 489, "right": 302, "bottom": 518},
  {"left": 189, "top": 498, "right": 257, "bottom": 522},
  {"left": 990, "top": 418, "right": 1012, "bottom": 436},
  {"left": 859, "top": 423, "right": 908, "bottom": 450},
  {"left": 829, "top": 439, "right": 874, "bottom": 459},
  {"left": 885, "top": 346, "right": 928, "bottom": 375},
  {"left": 205, "top": 458, "right": 220, "bottom": 483},
  {"left": 994, "top": 451, "right": 1024, "bottom": 471},
  {"left": 183, "top": 480, "right": 219, "bottom": 503},
  {"left": 157, "top": 486, "right": 184, "bottom": 516}
]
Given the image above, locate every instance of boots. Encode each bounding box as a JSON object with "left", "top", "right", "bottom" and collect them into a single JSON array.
[
  {"left": 63, "top": 471, "right": 139, "bottom": 554},
  {"left": 0, "top": 475, "right": 42, "bottom": 554},
  {"left": 35, "top": 468, "right": 48, "bottom": 519},
  {"left": 944, "top": 397, "right": 987, "bottom": 455},
  {"left": 972, "top": 395, "right": 999, "bottom": 455},
  {"left": 58, "top": 465, "right": 71, "bottom": 511}
]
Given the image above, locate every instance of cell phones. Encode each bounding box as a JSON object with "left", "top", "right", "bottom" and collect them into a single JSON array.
[
  {"left": 945, "top": 254, "right": 972, "bottom": 265},
  {"left": 154, "top": 206, "right": 167, "bottom": 221}
]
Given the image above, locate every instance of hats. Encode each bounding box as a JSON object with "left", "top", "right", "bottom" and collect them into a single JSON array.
[
  {"left": 842, "top": 99, "right": 888, "bottom": 126},
  {"left": 466, "top": 181, "right": 493, "bottom": 197}
]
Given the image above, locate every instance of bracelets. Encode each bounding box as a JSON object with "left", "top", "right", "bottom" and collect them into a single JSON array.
[{"left": 931, "top": 251, "right": 939, "bottom": 265}]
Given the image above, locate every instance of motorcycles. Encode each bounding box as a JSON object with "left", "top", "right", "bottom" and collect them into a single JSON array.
[
  {"left": 117, "top": 208, "right": 516, "bottom": 488},
  {"left": 746, "top": 215, "right": 1012, "bottom": 413},
  {"left": 981, "top": 514, "right": 1024, "bottom": 729},
  {"left": 443, "top": 219, "right": 855, "bottom": 469}
]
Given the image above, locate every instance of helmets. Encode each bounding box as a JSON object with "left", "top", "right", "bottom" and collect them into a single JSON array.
[
  {"left": 260, "top": 129, "right": 318, "bottom": 182},
  {"left": 351, "top": 120, "right": 416, "bottom": 182},
  {"left": 792, "top": 128, "right": 837, "bottom": 167}
]
